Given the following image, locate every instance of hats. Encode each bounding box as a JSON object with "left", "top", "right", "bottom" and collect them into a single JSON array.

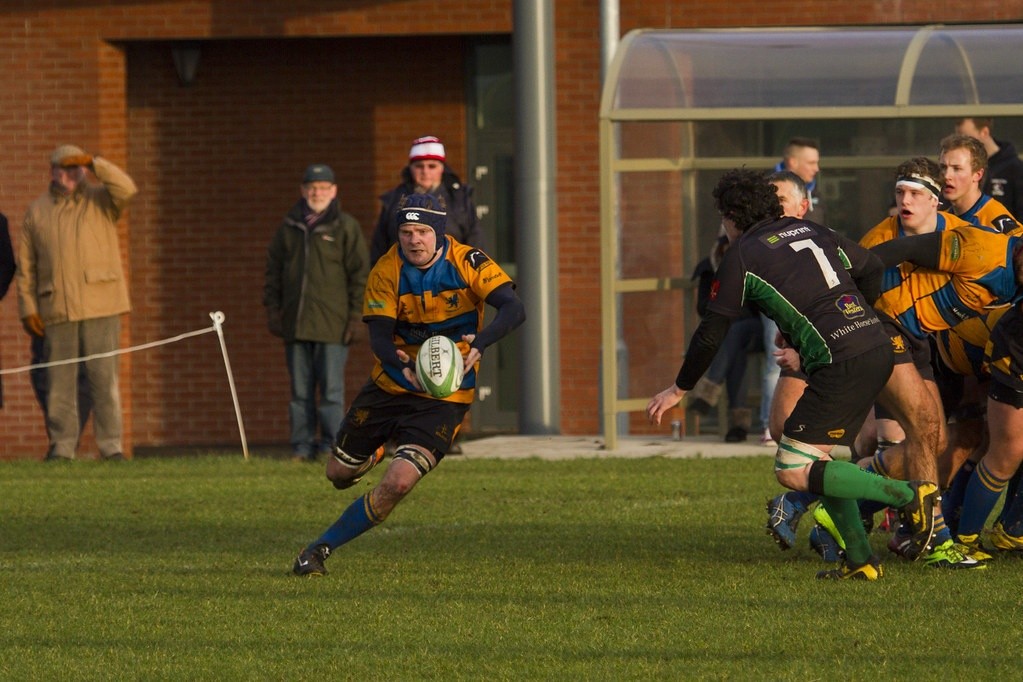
[
  {"left": 302, "top": 164, "right": 334, "bottom": 183},
  {"left": 409, "top": 136, "right": 447, "bottom": 164},
  {"left": 51, "top": 146, "right": 83, "bottom": 164}
]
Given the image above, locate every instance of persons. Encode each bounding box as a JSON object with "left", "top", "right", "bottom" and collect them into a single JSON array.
[
  {"left": 14, "top": 145, "right": 142, "bottom": 463},
  {"left": 371, "top": 137, "right": 488, "bottom": 455},
  {"left": 292, "top": 182, "right": 529, "bottom": 576},
  {"left": 695, "top": 117, "right": 1023, "bottom": 582},
  {"left": 0, "top": 210, "right": 19, "bottom": 411},
  {"left": 262, "top": 164, "right": 370, "bottom": 460},
  {"left": 647, "top": 164, "right": 941, "bottom": 582}
]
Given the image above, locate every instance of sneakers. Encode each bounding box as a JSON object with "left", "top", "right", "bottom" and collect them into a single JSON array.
[
  {"left": 865, "top": 506, "right": 1023, "bottom": 569},
  {"left": 815, "top": 555, "right": 882, "bottom": 581},
  {"left": 332, "top": 446, "right": 384, "bottom": 489},
  {"left": 293, "top": 544, "right": 332, "bottom": 577},
  {"left": 810, "top": 526, "right": 845, "bottom": 565},
  {"left": 814, "top": 501, "right": 848, "bottom": 549},
  {"left": 897, "top": 480, "right": 939, "bottom": 561},
  {"left": 766, "top": 492, "right": 805, "bottom": 551}
]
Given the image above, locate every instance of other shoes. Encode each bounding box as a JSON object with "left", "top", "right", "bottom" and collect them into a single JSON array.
[
  {"left": 46, "top": 454, "right": 70, "bottom": 467},
  {"left": 293, "top": 452, "right": 310, "bottom": 461},
  {"left": 686, "top": 399, "right": 708, "bottom": 416},
  {"left": 109, "top": 453, "right": 126, "bottom": 460},
  {"left": 763, "top": 428, "right": 776, "bottom": 446},
  {"left": 724, "top": 426, "right": 746, "bottom": 443}
]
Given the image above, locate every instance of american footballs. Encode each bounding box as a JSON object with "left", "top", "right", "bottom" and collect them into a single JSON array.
[{"left": 416, "top": 335, "right": 463, "bottom": 398}]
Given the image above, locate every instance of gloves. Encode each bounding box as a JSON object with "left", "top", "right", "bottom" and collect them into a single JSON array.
[
  {"left": 62, "top": 154, "right": 94, "bottom": 166},
  {"left": 342, "top": 320, "right": 367, "bottom": 347},
  {"left": 267, "top": 311, "right": 284, "bottom": 339},
  {"left": 24, "top": 314, "right": 44, "bottom": 337}
]
